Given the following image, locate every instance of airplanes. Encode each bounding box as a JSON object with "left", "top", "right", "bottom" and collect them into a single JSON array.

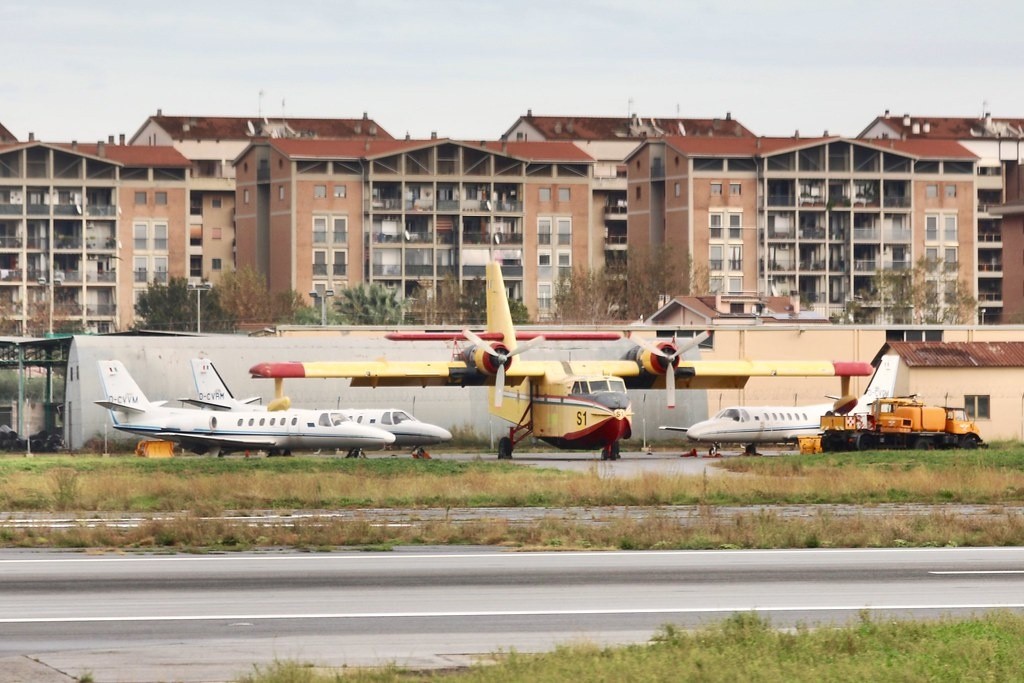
[
  {"left": 659, "top": 353, "right": 899, "bottom": 457},
  {"left": 250, "top": 261, "right": 875, "bottom": 460},
  {"left": 94, "top": 355, "right": 455, "bottom": 459}
]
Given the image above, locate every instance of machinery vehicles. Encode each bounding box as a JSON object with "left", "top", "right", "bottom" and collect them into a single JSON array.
[{"left": 821, "top": 395, "right": 987, "bottom": 451}]
